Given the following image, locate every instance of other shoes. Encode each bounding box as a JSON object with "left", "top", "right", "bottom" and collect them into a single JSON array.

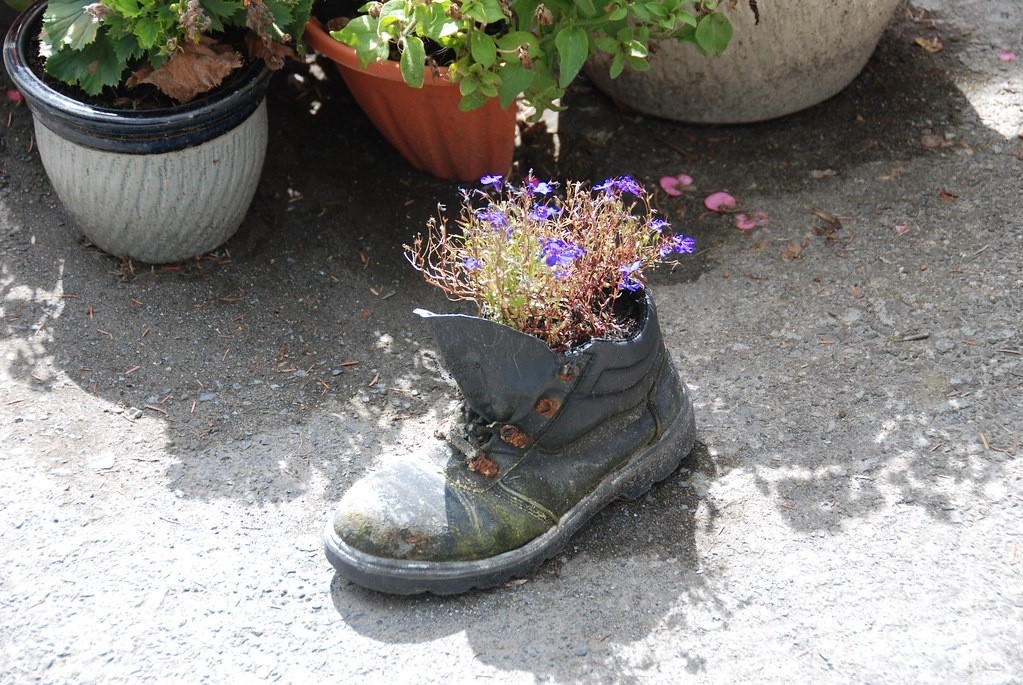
[{"left": 323, "top": 284, "right": 696, "bottom": 595}]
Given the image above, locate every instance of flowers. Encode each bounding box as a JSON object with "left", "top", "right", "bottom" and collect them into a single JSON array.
[
  {"left": 330, "top": 0, "right": 732, "bottom": 124},
  {"left": 37, "top": 0, "right": 317, "bottom": 102},
  {"left": 402, "top": 167, "right": 695, "bottom": 346}
]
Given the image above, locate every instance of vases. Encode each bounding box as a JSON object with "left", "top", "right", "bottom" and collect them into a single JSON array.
[
  {"left": 583, "top": 0, "right": 897, "bottom": 125},
  {"left": 3, "top": 0, "right": 268, "bottom": 265},
  {"left": 301, "top": 3, "right": 516, "bottom": 180}
]
